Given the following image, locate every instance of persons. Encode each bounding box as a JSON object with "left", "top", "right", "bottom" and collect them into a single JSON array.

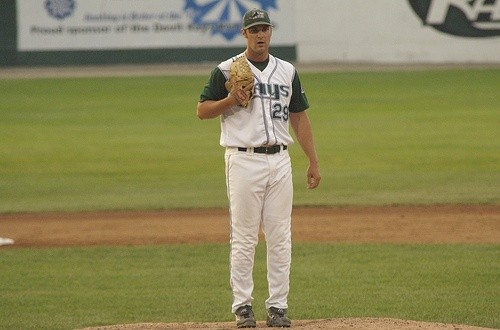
[{"left": 196, "top": 7, "right": 322, "bottom": 327}]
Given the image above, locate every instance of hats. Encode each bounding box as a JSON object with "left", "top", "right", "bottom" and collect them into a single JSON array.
[{"left": 242, "top": 8, "right": 272, "bottom": 30}]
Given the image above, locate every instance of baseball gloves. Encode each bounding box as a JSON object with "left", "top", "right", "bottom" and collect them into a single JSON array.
[{"left": 224, "top": 55, "right": 256, "bottom": 109}]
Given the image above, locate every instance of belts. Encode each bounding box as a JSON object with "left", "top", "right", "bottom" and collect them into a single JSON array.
[{"left": 238, "top": 145, "right": 288, "bottom": 154}]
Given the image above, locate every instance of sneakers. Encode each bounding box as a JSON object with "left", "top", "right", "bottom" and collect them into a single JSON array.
[
  {"left": 236, "top": 305, "right": 256, "bottom": 328},
  {"left": 266, "top": 306, "right": 292, "bottom": 327}
]
